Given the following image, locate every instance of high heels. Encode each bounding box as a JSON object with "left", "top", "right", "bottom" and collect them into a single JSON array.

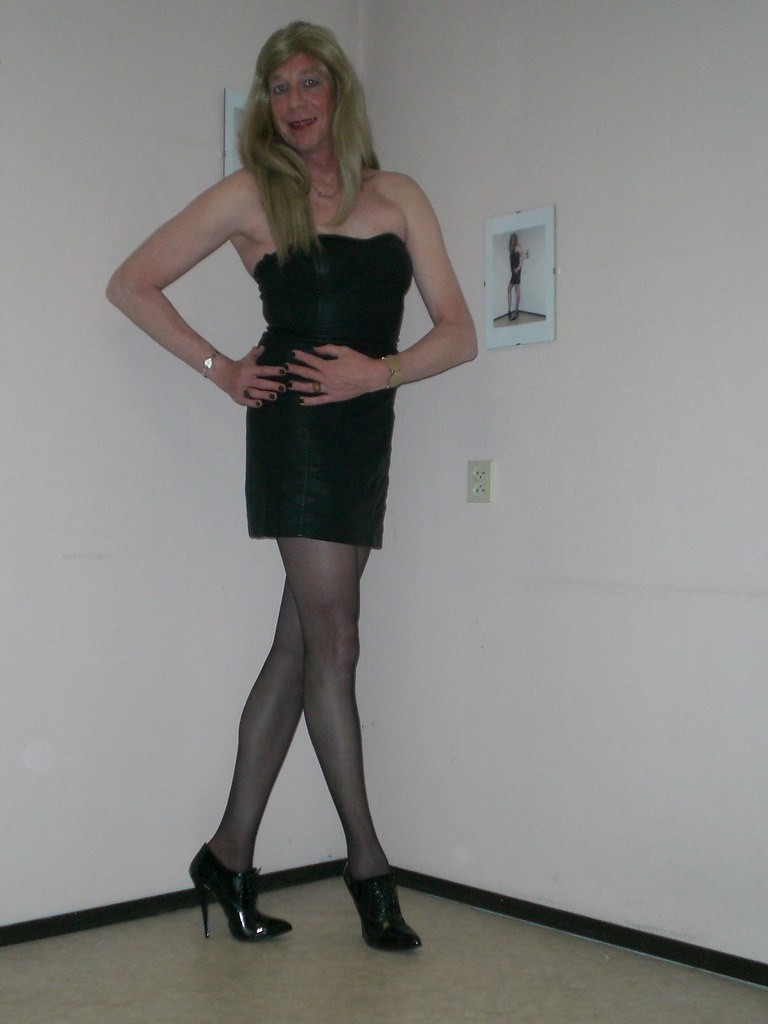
[
  {"left": 342, "top": 862, "right": 422, "bottom": 951},
  {"left": 189, "top": 842, "right": 291, "bottom": 942}
]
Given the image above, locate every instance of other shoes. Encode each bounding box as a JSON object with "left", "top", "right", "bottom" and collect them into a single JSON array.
[{"left": 508, "top": 311, "right": 520, "bottom": 321}]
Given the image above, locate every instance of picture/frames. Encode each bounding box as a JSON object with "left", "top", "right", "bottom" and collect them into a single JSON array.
[
  {"left": 222, "top": 87, "right": 249, "bottom": 178},
  {"left": 483, "top": 203, "right": 557, "bottom": 350}
]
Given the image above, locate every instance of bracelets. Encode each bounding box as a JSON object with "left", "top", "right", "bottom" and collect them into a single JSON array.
[
  {"left": 382, "top": 355, "right": 402, "bottom": 390},
  {"left": 203, "top": 350, "right": 220, "bottom": 378}
]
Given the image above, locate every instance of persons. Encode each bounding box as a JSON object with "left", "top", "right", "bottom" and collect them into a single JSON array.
[
  {"left": 507, "top": 233, "right": 525, "bottom": 321},
  {"left": 106, "top": 21, "right": 479, "bottom": 949}
]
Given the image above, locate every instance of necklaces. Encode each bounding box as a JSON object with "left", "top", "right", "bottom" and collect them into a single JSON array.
[{"left": 310, "top": 167, "right": 343, "bottom": 198}]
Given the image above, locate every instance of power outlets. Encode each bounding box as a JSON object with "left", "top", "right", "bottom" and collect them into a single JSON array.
[{"left": 466, "top": 459, "right": 492, "bottom": 504}]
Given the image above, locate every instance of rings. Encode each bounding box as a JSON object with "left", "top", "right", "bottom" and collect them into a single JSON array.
[
  {"left": 243, "top": 389, "right": 250, "bottom": 399},
  {"left": 312, "top": 382, "right": 321, "bottom": 394}
]
